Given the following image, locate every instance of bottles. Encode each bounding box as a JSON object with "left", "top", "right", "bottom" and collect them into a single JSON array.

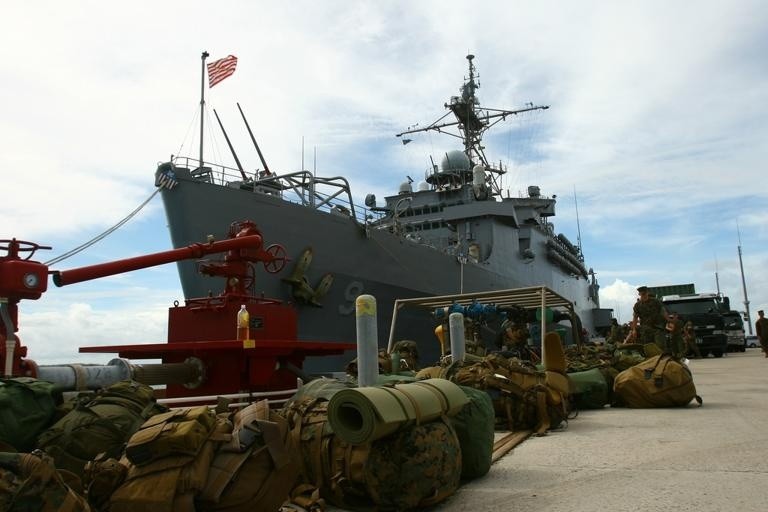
[{"left": 236, "top": 304, "right": 250, "bottom": 341}]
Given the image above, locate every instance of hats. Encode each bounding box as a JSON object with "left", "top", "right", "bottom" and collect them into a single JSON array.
[{"left": 637, "top": 286, "right": 648, "bottom": 293}]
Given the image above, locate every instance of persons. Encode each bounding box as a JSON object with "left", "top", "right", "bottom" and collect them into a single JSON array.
[
  {"left": 607, "top": 312, "right": 695, "bottom": 359},
  {"left": 632, "top": 286, "right": 670, "bottom": 353},
  {"left": 755, "top": 310, "right": 767, "bottom": 358}
]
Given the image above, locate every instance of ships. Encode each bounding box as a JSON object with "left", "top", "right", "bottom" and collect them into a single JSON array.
[{"left": 154, "top": 50, "right": 614, "bottom": 384}]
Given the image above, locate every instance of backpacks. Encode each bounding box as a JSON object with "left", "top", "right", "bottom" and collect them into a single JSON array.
[
  {"left": 0, "top": 335, "right": 496, "bottom": 512},
  {"left": 445, "top": 335, "right": 702, "bottom": 436}
]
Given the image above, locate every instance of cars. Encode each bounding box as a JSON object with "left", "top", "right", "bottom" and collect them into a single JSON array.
[{"left": 745, "top": 335, "right": 761, "bottom": 348}]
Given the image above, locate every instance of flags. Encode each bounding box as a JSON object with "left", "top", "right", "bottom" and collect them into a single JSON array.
[{"left": 204, "top": 54, "right": 237, "bottom": 88}]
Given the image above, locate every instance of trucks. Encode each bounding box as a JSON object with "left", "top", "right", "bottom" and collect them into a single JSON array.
[{"left": 647, "top": 284, "right": 744, "bottom": 358}]
